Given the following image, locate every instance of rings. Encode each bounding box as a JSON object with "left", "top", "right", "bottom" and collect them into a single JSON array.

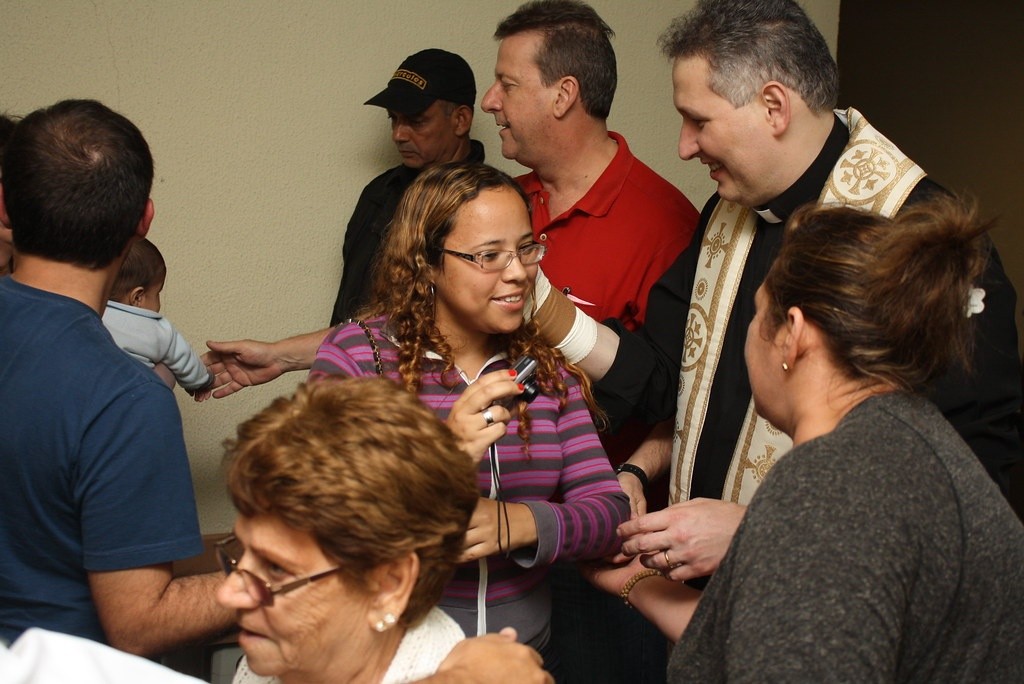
[
  {"left": 482, "top": 409, "right": 495, "bottom": 427},
  {"left": 664, "top": 551, "right": 673, "bottom": 568}
]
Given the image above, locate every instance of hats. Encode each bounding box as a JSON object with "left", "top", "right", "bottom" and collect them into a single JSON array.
[{"left": 363, "top": 49, "right": 476, "bottom": 114}]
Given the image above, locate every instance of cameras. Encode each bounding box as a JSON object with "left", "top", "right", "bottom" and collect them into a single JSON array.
[{"left": 491, "top": 354, "right": 539, "bottom": 410}]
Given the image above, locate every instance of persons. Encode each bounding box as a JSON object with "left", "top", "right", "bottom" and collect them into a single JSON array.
[
  {"left": 583, "top": 197, "right": 1024, "bottom": 684},
  {"left": 329, "top": 48, "right": 485, "bottom": 327},
  {"left": 0, "top": 376, "right": 557, "bottom": 684},
  {"left": 0, "top": 98, "right": 241, "bottom": 664},
  {"left": 522, "top": 0, "right": 1024, "bottom": 592},
  {"left": 198, "top": 0, "right": 700, "bottom": 684},
  {"left": 305, "top": 161, "right": 630, "bottom": 654}
]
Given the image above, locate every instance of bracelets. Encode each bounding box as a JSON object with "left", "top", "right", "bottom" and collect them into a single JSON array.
[
  {"left": 615, "top": 463, "right": 648, "bottom": 488},
  {"left": 620, "top": 570, "right": 662, "bottom": 609}
]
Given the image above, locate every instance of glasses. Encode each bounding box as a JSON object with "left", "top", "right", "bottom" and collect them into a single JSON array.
[
  {"left": 433, "top": 240, "right": 548, "bottom": 271},
  {"left": 213, "top": 534, "right": 349, "bottom": 606}
]
